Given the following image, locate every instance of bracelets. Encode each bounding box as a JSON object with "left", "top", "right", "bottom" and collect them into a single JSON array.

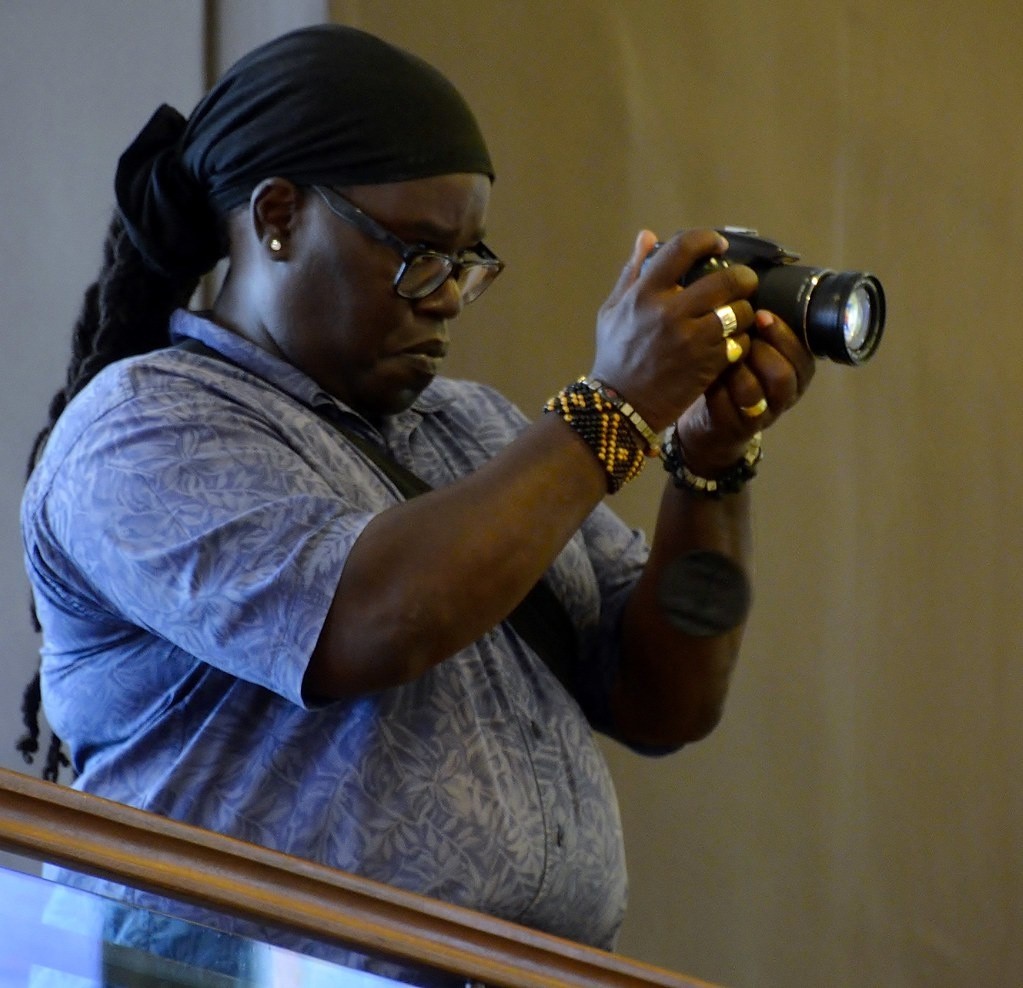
[
  {"left": 660, "top": 422, "right": 763, "bottom": 499},
  {"left": 543, "top": 376, "right": 661, "bottom": 495}
]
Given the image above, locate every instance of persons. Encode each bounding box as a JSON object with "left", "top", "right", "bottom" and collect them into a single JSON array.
[{"left": 13, "top": 22, "right": 815, "bottom": 988}]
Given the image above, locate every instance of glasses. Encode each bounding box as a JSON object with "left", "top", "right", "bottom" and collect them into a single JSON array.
[{"left": 309, "top": 183, "right": 506, "bottom": 305}]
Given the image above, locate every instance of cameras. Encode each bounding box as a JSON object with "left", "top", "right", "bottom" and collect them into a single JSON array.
[{"left": 638, "top": 225, "right": 887, "bottom": 367}]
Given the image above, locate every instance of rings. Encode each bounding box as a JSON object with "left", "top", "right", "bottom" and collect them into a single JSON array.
[
  {"left": 726, "top": 338, "right": 742, "bottom": 363},
  {"left": 740, "top": 398, "right": 768, "bottom": 417},
  {"left": 714, "top": 305, "right": 737, "bottom": 339}
]
